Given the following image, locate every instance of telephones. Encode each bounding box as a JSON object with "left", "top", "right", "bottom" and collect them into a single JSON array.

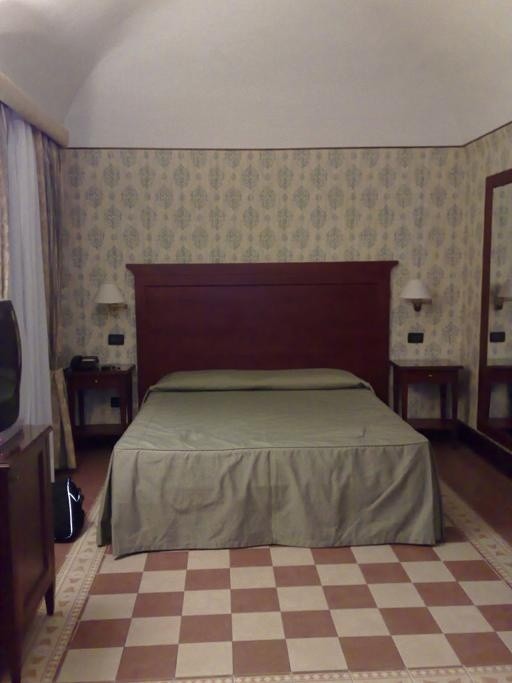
[{"left": 70, "top": 355, "right": 99, "bottom": 370}]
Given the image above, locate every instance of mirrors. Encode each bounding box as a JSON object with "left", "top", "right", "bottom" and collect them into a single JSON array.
[{"left": 478, "top": 167, "right": 511, "bottom": 452}]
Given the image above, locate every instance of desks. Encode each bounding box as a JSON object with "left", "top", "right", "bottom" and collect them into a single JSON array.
[
  {"left": 61, "top": 361, "right": 136, "bottom": 450},
  {"left": 2, "top": 422, "right": 59, "bottom": 683},
  {"left": 487, "top": 358, "right": 511, "bottom": 434},
  {"left": 388, "top": 357, "right": 465, "bottom": 439}
]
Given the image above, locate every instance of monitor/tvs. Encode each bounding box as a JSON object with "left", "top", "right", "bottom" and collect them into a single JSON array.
[{"left": 0, "top": 301, "right": 22, "bottom": 433}]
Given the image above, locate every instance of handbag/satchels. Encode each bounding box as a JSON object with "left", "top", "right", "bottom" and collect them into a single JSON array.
[{"left": 51, "top": 476, "right": 85, "bottom": 543}]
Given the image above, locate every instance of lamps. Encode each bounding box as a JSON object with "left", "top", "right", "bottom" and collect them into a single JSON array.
[
  {"left": 95, "top": 281, "right": 127, "bottom": 310},
  {"left": 492, "top": 278, "right": 510, "bottom": 310},
  {"left": 399, "top": 277, "right": 434, "bottom": 311}
]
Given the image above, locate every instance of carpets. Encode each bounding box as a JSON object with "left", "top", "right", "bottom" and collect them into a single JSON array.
[{"left": 20, "top": 460, "right": 511, "bottom": 683}]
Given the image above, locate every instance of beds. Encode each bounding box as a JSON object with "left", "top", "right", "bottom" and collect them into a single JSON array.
[{"left": 95, "top": 262, "right": 447, "bottom": 557}]
